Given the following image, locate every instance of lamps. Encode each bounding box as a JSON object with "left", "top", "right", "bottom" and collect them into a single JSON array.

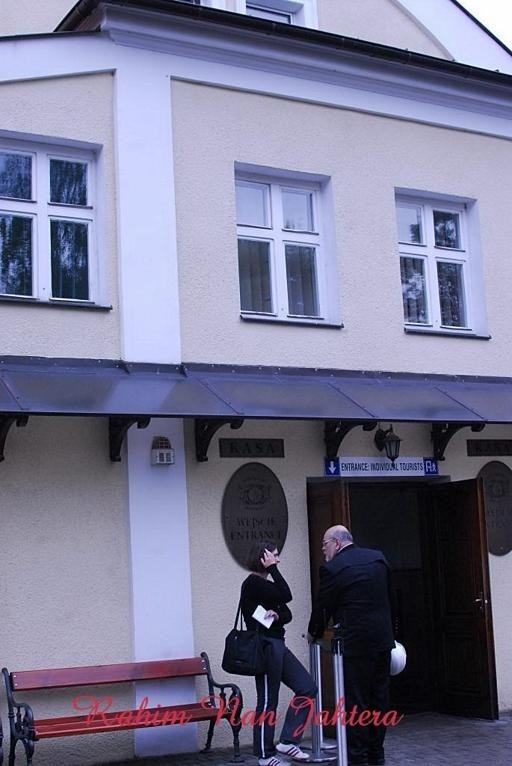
[{"left": 374, "top": 422, "right": 403, "bottom": 470}]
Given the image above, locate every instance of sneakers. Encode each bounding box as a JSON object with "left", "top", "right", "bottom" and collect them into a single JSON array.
[
  {"left": 274, "top": 738, "right": 311, "bottom": 761},
  {"left": 257, "top": 755, "right": 292, "bottom": 766}
]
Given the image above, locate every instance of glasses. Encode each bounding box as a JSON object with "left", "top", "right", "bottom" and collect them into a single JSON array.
[{"left": 321, "top": 537, "right": 334, "bottom": 547}]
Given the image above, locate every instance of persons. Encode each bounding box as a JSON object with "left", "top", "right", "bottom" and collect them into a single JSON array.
[
  {"left": 307, "top": 523, "right": 402, "bottom": 766},
  {"left": 240, "top": 539, "right": 317, "bottom": 766}
]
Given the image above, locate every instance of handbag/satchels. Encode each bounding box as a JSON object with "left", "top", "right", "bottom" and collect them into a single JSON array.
[{"left": 221, "top": 627, "right": 266, "bottom": 677}]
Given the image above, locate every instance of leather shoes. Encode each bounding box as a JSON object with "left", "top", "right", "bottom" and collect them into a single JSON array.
[
  {"left": 328, "top": 751, "right": 372, "bottom": 766},
  {"left": 369, "top": 745, "right": 386, "bottom": 765}
]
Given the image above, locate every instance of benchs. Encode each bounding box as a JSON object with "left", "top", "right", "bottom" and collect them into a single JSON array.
[{"left": 1, "top": 651, "right": 244, "bottom": 765}]
